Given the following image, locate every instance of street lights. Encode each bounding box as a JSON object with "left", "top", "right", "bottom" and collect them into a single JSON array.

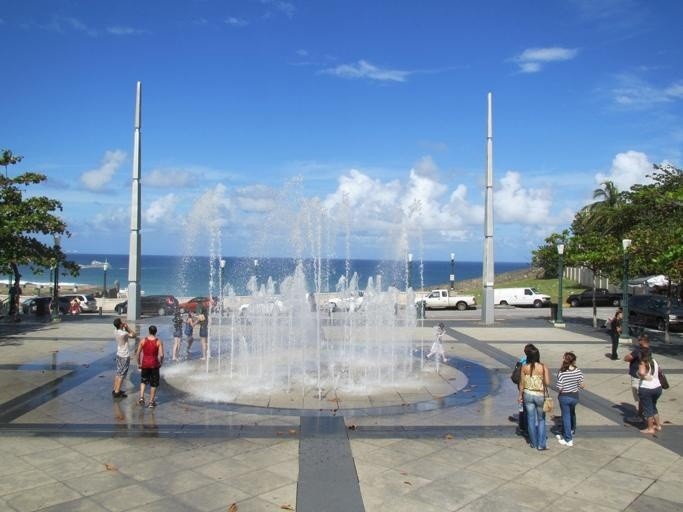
[
  {"left": 555, "top": 241, "right": 565, "bottom": 324},
  {"left": 101, "top": 257, "right": 109, "bottom": 298},
  {"left": 407, "top": 252, "right": 413, "bottom": 289},
  {"left": 448, "top": 252, "right": 455, "bottom": 291},
  {"left": 51, "top": 234, "right": 63, "bottom": 321},
  {"left": 219, "top": 259, "right": 226, "bottom": 316},
  {"left": 252, "top": 257, "right": 259, "bottom": 290},
  {"left": 621, "top": 238, "right": 631, "bottom": 340}
]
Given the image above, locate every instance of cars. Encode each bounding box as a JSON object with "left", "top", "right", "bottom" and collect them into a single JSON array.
[
  {"left": 619, "top": 295, "right": 682, "bottom": 332},
  {"left": 238, "top": 295, "right": 286, "bottom": 317},
  {"left": 178, "top": 297, "right": 221, "bottom": 315},
  {"left": 323, "top": 291, "right": 368, "bottom": 313}
]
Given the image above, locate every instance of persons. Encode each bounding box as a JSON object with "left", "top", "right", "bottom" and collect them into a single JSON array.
[
  {"left": 636, "top": 347, "right": 663, "bottom": 435},
  {"left": 136, "top": 325, "right": 165, "bottom": 408},
  {"left": 555, "top": 352, "right": 585, "bottom": 447},
  {"left": 611, "top": 311, "right": 624, "bottom": 360},
  {"left": 69, "top": 299, "right": 82, "bottom": 321},
  {"left": 426, "top": 322, "right": 451, "bottom": 362},
  {"left": 7, "top": 282, "right": 23, "bottom": 314},
  {"left": 170, "top": 306, "right": 208, "bottom": 361},
  {"left": 516, "top": 343, "right": 536, "bottom": 434},
  {"left": 517, "top": 347, "right": 551, "bottom": 451},
  {"left": 624, "top": 332, "right": 650, "bottom": 415},
  {"left": 112, "top": 318, "right": 137, "bottom": 398}
]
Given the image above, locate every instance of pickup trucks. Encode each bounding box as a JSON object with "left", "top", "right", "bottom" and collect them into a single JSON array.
[
  {"left": 566, "top": 288, "right": 631, "bottom": 307},
  {"left": 414, "top": 288, "right": 477, "bottom": 311}
]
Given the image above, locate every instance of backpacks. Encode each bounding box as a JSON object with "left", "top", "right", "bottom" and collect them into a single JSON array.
[{"left": 511, "top": 362, "right": 521, "bottom": 383}]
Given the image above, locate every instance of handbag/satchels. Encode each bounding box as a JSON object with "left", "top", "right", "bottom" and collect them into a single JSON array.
[
  {"left": 542, "top": 398, "right": 553, "bottom": 412},
  {"left": 658, "top": 371, "right": 670, "bottom": 389}
]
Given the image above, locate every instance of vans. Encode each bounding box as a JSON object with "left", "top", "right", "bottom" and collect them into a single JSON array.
[
  {"left": 493, "top": 288, "right": 551, "bottom": 307},
  {"left": 60, "top": 293, "right": 96, "bottom": 312},
  {"left": 22, "top": 296, "right": 70, "bottom": 314},
  {"left": 114, "top": 295, "right": 179, "bottom": 315}
]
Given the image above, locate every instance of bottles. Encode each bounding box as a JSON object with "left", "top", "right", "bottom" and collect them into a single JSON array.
[{"left": 518, "top": 400, "right": 523, "bottom": 412}]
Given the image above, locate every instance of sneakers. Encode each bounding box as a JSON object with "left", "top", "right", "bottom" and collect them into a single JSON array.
[
  {"left": 556, "top": 432, "right": 563, "bottom": 439},
  {"left": 641, "top": 429, "right": 654, "bottom": 434},
  {"left": 148, "top": 401, "right": 156, "bottom": 408},
  {"left": 112, "top": 391, "right": 127, "bottom": 397},
  {"left": 138, "top": 397, "right": 145, "bottom": 403},
  {"left": 559, "top": 438, "right": 573, "bottom": 446},
  {"left": 653, "top": 425, "right": 661, "bottom": 431}
]
